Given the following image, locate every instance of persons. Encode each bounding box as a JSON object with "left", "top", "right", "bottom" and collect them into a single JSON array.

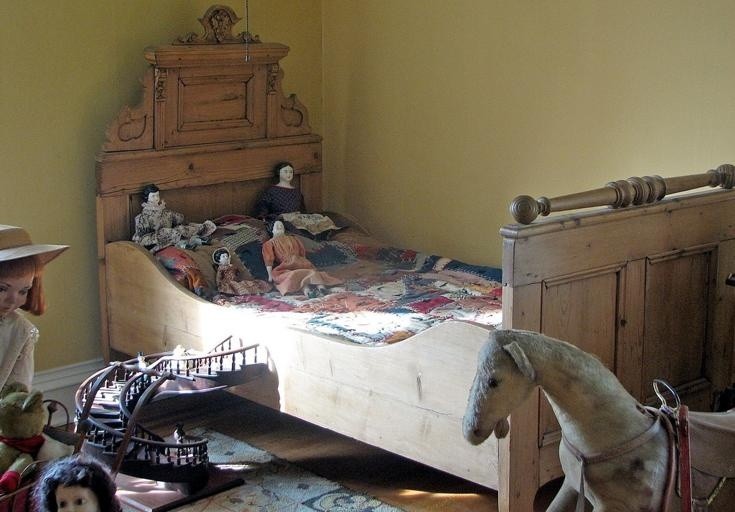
[
  {"left": 132, "top": 184, "right": 217, "bottom": 252},
  {"left": 262, "top": 214, "right": 342, "bottom": 298},
  {"left": 37, "top": 458, "right": 123, "bottom": 512},
  {"left": 256, "top": 161, "right": 342, "bottom": 240},
  {"left": 212, "top": 247, "right": 272, "bottom": 296},
  {"left": 0, "top": 225, "right": 72, "bottom": 394}
]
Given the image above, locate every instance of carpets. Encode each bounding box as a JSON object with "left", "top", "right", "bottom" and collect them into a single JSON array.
[{"left": 111, "top": 423, "right": 401, "bottom": 509}]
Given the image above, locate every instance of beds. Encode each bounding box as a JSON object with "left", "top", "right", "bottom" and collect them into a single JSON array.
[{"left": 91, "top": 4, "right": 733, "bottom": 511}]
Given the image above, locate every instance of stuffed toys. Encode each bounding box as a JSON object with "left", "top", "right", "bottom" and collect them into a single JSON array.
[{"left": 0, "top": 382, "right": 50, "bottom": 512}]
